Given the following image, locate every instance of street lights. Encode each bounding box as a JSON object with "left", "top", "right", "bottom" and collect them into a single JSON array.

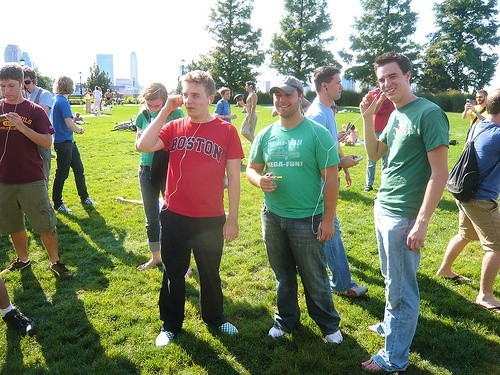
[
  {"left": 181, "top": 59, "right": 186, "bottom": 73},
  {"left": 79, "top": 71, "right": 83, "bottom": 94}
]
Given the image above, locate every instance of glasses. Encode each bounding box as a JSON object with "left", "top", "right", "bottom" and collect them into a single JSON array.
[{"left": 25, "top": 80, "right": 34, "bottom": 84}]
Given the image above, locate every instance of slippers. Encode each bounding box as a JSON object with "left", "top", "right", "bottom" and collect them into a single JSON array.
[
  {"left": 435, "top": 272, "right": 471, "bottom": 283},
  {"left": 475, "top": 296, "right": 500, "bottom": 314}
]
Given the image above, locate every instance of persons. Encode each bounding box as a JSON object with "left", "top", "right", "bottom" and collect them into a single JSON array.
[
  {"left": 134, "top": 50, "right": 500, "bottom": 371},
  {"left": 0, "top": 63, "right": 119, "bottom": 337}
]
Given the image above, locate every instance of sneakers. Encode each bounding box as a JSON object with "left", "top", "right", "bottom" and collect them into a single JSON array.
[
  {"left": 2, "top": 304, "right": 36, "bottom": 335},
  {"left": 50, "top": 261, "right": 70, "bottom": 278},
  {"left": 341, "top": 285, "right": 369, "bottom": 297},
  {"left": 6, "top": 258, "right": 31, "bottom": 272}
]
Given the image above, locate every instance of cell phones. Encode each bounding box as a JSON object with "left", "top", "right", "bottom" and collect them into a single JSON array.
[
  {"left": 466, "top": 98, "right": 470, "bottom": 104},
  {"left": 0, "top": 114, "right": 8, "bottom": 122}
]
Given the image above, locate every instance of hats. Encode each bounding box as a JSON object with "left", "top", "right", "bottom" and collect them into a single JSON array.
[{"left": 269, "top": 76, "right": 303, "bottom": 95}]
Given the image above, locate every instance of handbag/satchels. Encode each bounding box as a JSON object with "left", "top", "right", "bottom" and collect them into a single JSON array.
[{"left": 148, "top": 149, "right": 170, "bottom": 185}]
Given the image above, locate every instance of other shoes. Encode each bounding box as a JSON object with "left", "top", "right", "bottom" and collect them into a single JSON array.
[
  {"left": 81, "top": 197, "right": 96, "bottom": 205},
  {"left": 325, "top": 330, "right": 343, "bottom": 344},
  {"left": 268, "top": 327, "right": 286, "bottom": 338},
  {"left": 218, "top": 321, "right": 239, "bottom": 335},
  {"left": 54, "top": 204, "right": 72, "bottom": 212},
  {"left": 155, "top": 330, "right": 175, "bottom": 347}
]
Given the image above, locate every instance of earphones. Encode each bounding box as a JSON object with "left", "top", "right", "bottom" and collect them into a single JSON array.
[
  {"left": 208, "top": 99, "right": 211, "bottom": 106},
  {"left": 299, "top": 97, "right": 303, "bottom": 103},
  {"left": 20, "top": 83, "right": 23, "bottom": 89}
]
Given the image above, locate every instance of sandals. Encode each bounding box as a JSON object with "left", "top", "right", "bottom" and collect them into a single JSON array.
[{"left": 363, "top": 186, "right": 373, "bottom": 191}]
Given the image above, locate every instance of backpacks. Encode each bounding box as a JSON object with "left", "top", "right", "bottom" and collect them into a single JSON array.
[{"left": 446, "top": 122, "right": 500, "bottom": 202}]
